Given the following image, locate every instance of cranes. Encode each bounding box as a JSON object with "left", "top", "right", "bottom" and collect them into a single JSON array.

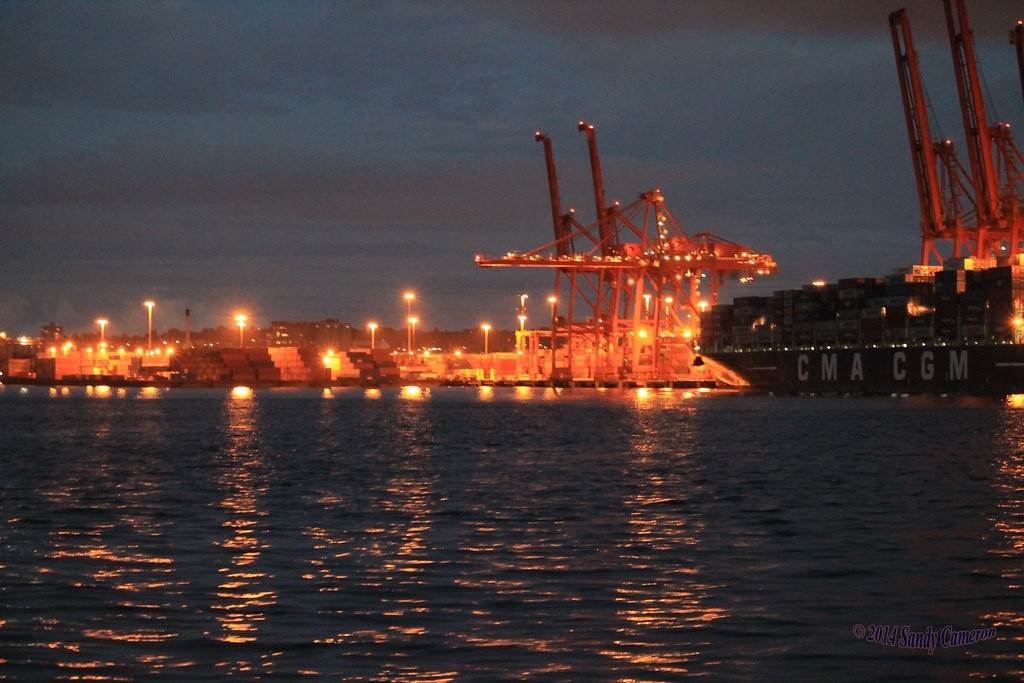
[
  {"left": 877, "top": 0, "right": 1024, "bottom": 276},
  {"left": 476, "top": 120, "right": 778, "bottom": 389}
]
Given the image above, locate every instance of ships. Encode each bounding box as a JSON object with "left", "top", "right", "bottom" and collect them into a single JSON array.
[{"left": 698, "top": 263, "right": 1024, "bottom": 396}]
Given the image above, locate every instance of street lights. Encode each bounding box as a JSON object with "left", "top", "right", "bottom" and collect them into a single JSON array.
[
  {"left": 98, "top": 319, "right": 108, "bottom": 341},
  {"left": 368, "top": 323, "right": 378, "bottom": 349},
  {"left": 405, "top": 293, "right": 415, "bottom": 352},
  {"left": 548, "top": 296, "right": 557, "bottom": 330},
  {"left": 409, "top": 318, "right": 417, "bottom": 350},
  {"left": 144, "top": 301, "right": 155, "bottom": 363},
  {"left": 480, "top": 325, "right": 491, "bottom": 355},
  {"left": 236, "top": 313, "right": 248, "bottom": 347}
]
